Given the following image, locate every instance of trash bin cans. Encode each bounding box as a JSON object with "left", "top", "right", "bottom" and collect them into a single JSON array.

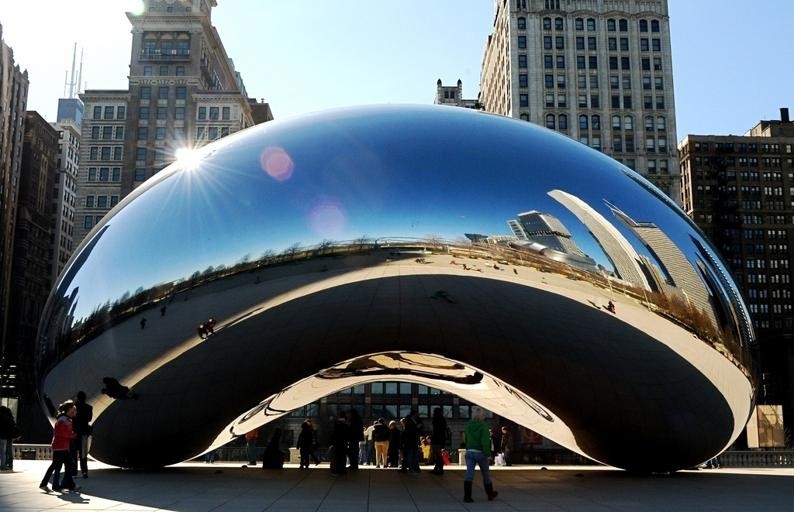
[
  {"left": 459, "top": 449, "right": 466, "bottom": 465},
  {"left": 290, "top": 448, "right": 301, "bottom": 464}
]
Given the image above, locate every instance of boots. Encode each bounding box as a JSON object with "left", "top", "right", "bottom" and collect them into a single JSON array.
[
  {"left": 463, "top": 481, "right": 474, "bottom": 503},
  {"left": 486, "top": 482, "right": 498, "bottom": 501}
]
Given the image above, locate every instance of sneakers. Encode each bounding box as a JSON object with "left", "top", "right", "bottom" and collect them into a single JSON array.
[
  {"left": 434, "top": 468, "right": 444, "bottom": 474},
  {"left": 401, "top": 467, "right": 420, "bottom": 473},
  {"left": 376, "top": 465, "right": 380, "bottom": 468},
  {"left": 206, "top": 459, "right": 215, "bottom": 463},
  {"left": 300, "top": 465, "right": 308, "bottom": 469},
  {"left": 247, "top": 461, "right": 257, "bottom": 465},
  {"left": 383, "top": 465, "right": 388, "bottom": 468},
  {"left": 39, "top": 472, "right": 87, "bottom": 494},
  {"left": 360, "top": 461, "right": 375, "bottom": 465},
  {"left": 0, "top": 464, "right": 13, "bottom": 472}
]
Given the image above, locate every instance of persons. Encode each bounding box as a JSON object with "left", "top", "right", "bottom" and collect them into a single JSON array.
[
  {"left": 0, "top": 399, "right": 82, "bottom": 493},
  {"left": 244, "top": 405, "right": 513, "bottom": 503},
  {"left": 160, "top": 305, "right": 166, "bottom": 316},
  {"left": 140, "top": 316, "right": 148, "bottom": 329},
  {"left": 705, "top": 457, "right": 720, "bottom": 469},
  {"left": 71, "top": 391, "right": 93, "bottom": 479},
  {"left": 207, "top": 316, "right": 216, "bottom": 334},
  {"left": 197, "top": 320, "right": 208, "bottom": 340},
  {"left": 100, "top": 376, "right": 138, "bottom": 401},
  {"left": 206, "top": 450, "right": 216, "bottom": 463},
  {"left": 608, "top": 301, "right": 616, "bottom": 314}
]
